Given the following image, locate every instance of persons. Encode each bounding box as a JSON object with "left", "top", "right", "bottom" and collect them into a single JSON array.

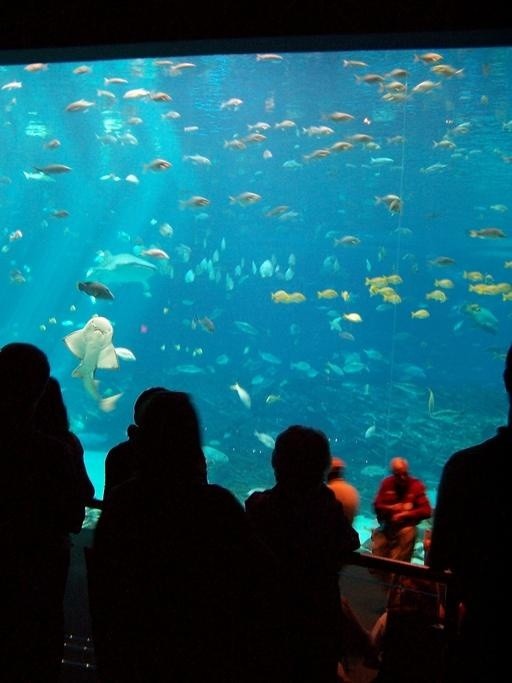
[
  {"left": 371, "top": 456, "right": 433, "bottom": 563},
  {"left": 0, "top": 343, "right": 95, "bottom": 683},
  {"left": 426, "top": 347, "right": 511, "bottom": 683},
  {"left": 363, "top": 574, "right": 444, "bottom": 683},
  {"left": 92, "top": 387, "right": 361, "bottom": 683},
  {"left": 326, "top": 456, "right": 359, "bottom": 524}
]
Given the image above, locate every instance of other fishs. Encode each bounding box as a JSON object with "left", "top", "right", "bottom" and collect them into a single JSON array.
[{"left": 1, "top": 50, "right": 512, "bottom": 560}]
